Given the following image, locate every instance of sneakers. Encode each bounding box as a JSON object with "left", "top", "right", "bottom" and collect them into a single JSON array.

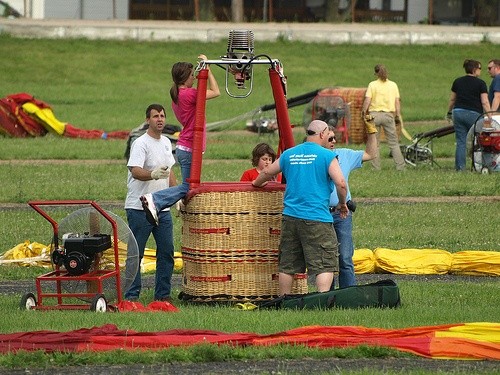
[{"left": 140, "top": 192, "right": 159, "bottom": 227}]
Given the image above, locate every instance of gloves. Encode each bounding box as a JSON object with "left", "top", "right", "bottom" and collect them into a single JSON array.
[
  {"left": 362, "top": 111, "right": 378, "bottom": 133},
  {"left": 446, "top": 111, "right": 453, "bottom": 123},
  {"left": 151, "top": 165, "right": 169, "bottom": 180},
  {"left": 394, "top": 114, "right": 401, "bottom": 126},
  {"left": 176, "top": 199, "right": 183, "bottom": 211}
]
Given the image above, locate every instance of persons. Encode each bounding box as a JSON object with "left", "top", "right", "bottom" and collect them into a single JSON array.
[
  {"left": 324, "top": 110, "right": 378, "bottom": 290},
  {"left": 139, "top": 55, "right": 221, "bottom": 227},
  {"left": 360, "top": 64, "right": 406, "bottom": 171},
  {"left": 488, "top": 59, "right": 500, "bottom": 172},
  {"left": 240, "top": 143, "right": 282, "bottom": 184},
  {"left": 251, "top": 119, "right": 349, "bottom": 297},
  {"left": 448, "top": 59, "right": 491, "bottom": 172},
  {"left": 124, "top": 103, "right": 182, "bottom": 303}
]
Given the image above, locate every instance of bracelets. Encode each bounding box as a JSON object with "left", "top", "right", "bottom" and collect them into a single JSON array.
[{"left": 447, "top": 111, "right": 453, "bottom": 116}]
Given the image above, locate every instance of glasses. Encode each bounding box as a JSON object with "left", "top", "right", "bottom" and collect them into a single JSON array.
[
  {"left": 476, "top": 66, "right": 481, "bottom": 69},
  {"left": 306, "top": 126, "right": 327, "bottom": 136},
  {"left": 488, "top": 67, "right": 491, "bottom": 70},
  {"left": 326, "top": 136, "right": 336, "bottom": 142}
]
